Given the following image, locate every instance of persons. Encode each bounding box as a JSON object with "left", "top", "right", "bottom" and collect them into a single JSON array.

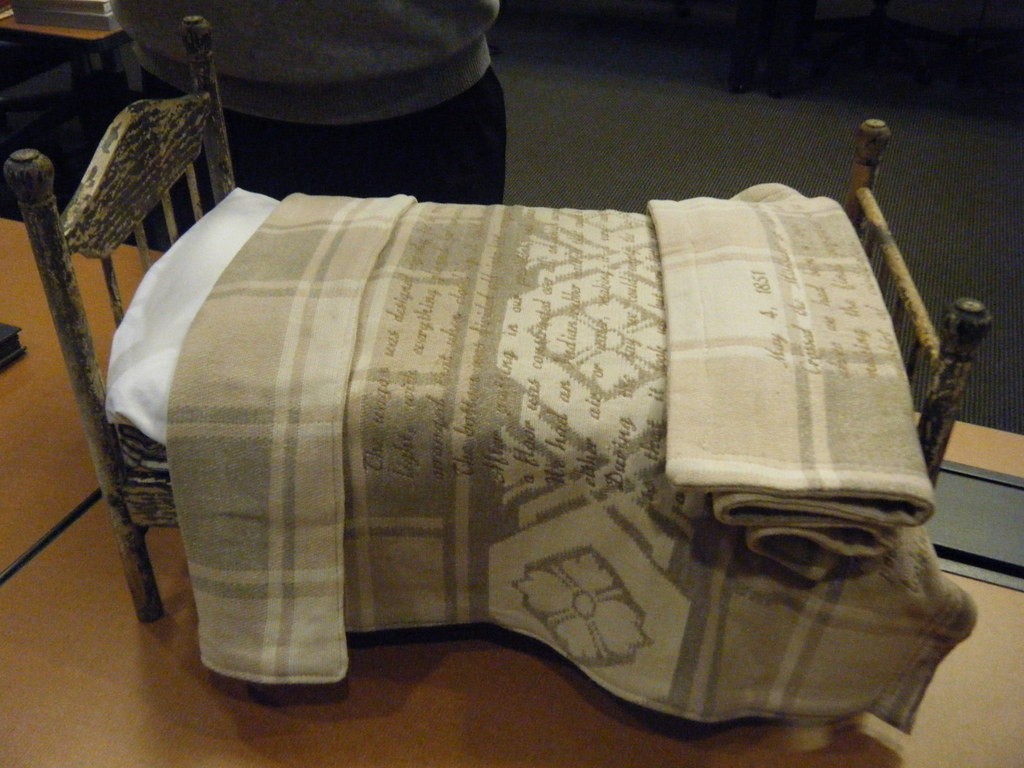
[{"left": 110, "top": 1, "right": 506, "bottom": 238}]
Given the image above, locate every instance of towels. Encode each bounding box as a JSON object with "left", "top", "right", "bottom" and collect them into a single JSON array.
[{"left": 101, "top": 187, "right": 977, "bottom": 738}]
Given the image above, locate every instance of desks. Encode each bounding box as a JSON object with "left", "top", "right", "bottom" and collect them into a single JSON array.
[{"left": 0, "top": 0, "right": 1024, "bottom": 768}]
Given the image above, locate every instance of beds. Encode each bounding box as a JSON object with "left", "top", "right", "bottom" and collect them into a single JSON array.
[{"left": 6, "top": 17, "right": 992, "bottom": 733}]
